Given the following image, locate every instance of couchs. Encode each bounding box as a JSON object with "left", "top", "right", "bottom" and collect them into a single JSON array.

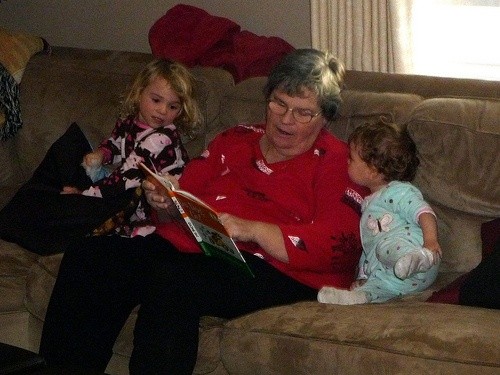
[{"left": 0, "top": 47, "right": 499, "bottom": 375}]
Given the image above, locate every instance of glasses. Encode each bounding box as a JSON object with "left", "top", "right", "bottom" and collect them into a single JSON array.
[{"left": 257, "top": 100, "right": 323, "bottom": 124}]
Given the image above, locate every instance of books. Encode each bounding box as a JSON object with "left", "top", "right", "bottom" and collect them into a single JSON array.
[{"left": 139, "top": 158, "right": 255, "bottom": 278}]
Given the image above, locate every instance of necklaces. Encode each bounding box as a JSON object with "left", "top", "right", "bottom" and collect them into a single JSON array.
[{"left": 264, "top": 142, "right": 288, "bottom": 164}]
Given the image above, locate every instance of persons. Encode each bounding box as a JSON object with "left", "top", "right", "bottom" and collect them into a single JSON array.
[
  {"left": 62, "top": 56, "right": 204, "bottom": 230},
  {"left": 318, "top": 119, "right": 443, "bottom": 306},
  {"left": 45, "top": 48, "right": 371, "bottom": 375}
]
